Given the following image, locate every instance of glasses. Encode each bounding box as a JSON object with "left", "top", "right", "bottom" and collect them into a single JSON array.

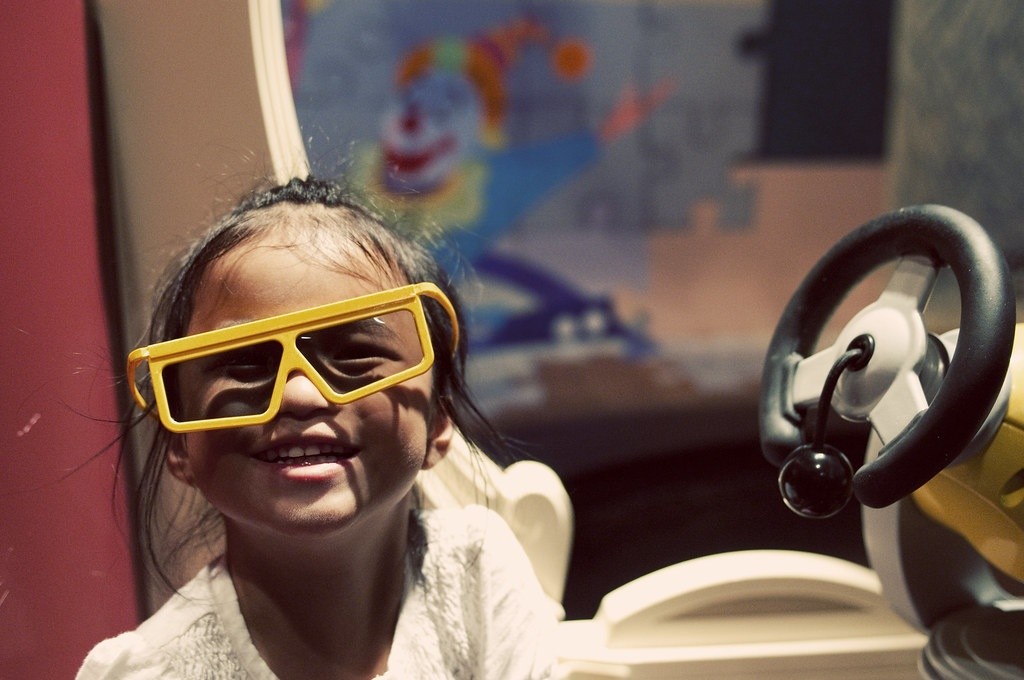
[{"left": 125, "top": 282, "right": 461, "bottom": 434}]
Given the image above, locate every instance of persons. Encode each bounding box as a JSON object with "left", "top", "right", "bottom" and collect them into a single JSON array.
[{"left": 71, "top": 174, "right": 569, "bottom": 680}]
